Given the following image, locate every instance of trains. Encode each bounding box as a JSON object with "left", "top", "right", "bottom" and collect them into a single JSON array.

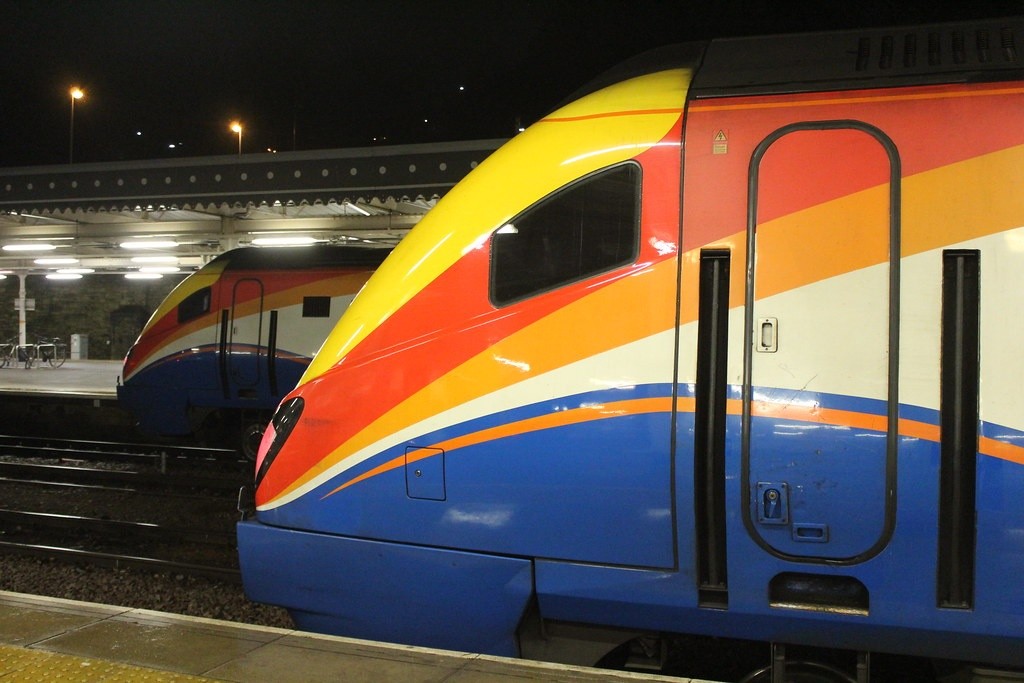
[
  {"left": 112, "top": 243, "right": 402, "bottom": 468},
  {"left": 234, "top": 65, "right": 1024, "bottom": 683}
]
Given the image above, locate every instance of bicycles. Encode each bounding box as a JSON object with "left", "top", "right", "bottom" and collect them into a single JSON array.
[
  {"left": 0, "top": 331, "right": 33, "bottom": 369},
  {"left": 24, "top": 331, "right": 68, "bottom": 370}
]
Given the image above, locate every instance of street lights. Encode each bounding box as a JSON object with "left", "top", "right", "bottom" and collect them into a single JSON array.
[
  {"left": 229, "top": 118, "right": 244, "bottom": 155},
  {"left": 66, "top": 85, "right": 85, "bottom": 164}
]
